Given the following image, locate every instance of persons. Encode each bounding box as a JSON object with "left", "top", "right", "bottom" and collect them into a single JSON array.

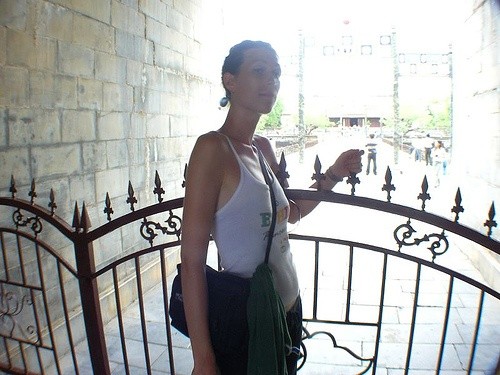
[
  {"left": 364, "top": 133, "right": 378, "bottom": 176},
  {"left": 409, "top": 133, "right": 448, "bottom": 188},
  {"left": 169, "top": 40, "right": 364, "bottom": 375}
]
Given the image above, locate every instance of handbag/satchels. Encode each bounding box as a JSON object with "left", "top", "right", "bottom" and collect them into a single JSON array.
[{"left": 169, "top": 263, "right": 250, "bottom": 358}]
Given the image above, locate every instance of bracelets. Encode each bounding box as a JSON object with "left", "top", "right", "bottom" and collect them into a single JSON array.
[{"left": 326, "top": 166, "right": 343, "bottom": 182}]
